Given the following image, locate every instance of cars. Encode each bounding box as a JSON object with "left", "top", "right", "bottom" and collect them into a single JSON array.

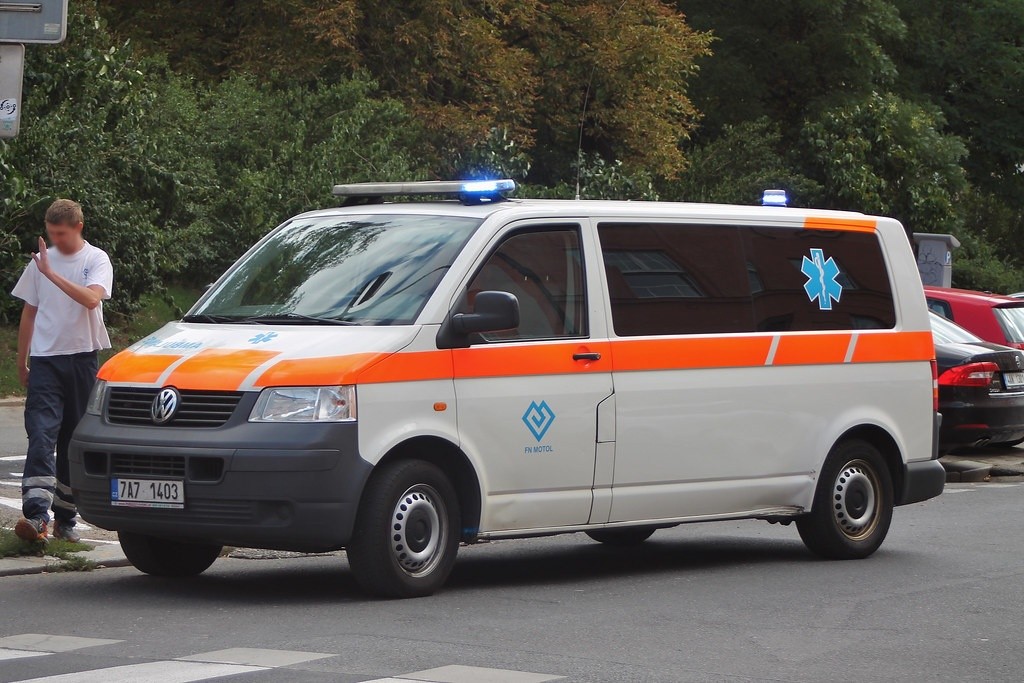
[
  {"left": 928, "top": 307, "right": 1024, "bottom": 455},
  {"left": 924, "top": 283, "right": 1024, "bottom": 350}
]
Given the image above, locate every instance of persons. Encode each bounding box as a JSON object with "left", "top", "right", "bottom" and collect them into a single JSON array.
[{"left": 11, "top": 198, "right": 114, "bottom": 550}]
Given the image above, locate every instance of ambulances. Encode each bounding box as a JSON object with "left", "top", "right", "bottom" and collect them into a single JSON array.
[{"left": 68, "top": 177, "right": 947, "bottom": 599}]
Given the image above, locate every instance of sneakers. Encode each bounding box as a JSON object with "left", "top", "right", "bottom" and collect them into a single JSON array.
[
  {"left": 15, "top": 516, "right": 49, "bottom": 554},
  {"left": 51, "top": 519, "right": 81, "bottom": 542}
]
